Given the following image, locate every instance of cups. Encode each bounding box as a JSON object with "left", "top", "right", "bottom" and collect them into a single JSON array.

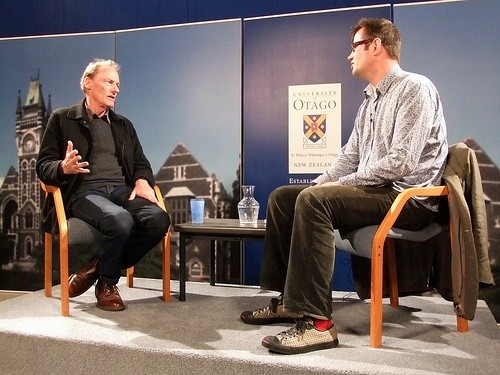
[{"left": 189, "top": 198, "right": 205, "bottom": 224}]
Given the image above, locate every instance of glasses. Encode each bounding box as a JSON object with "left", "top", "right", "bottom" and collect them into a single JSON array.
[{"left": 349, "top": 37, "right": 383, "bottom": 51}]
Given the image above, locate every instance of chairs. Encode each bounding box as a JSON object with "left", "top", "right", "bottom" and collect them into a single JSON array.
[
  {"left": 40, "top": 181, "right": 171, "bottom": 317},
  {"left": 334, "top": 142, "right": 479, "bottom": 347}
]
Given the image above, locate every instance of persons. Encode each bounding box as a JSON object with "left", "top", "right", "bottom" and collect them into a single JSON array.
[
  {"left": 241, "top": 17, "right": 450, "bottom": 356},
  {"left": 37, "top": 60, "right": 171, "bottom": 311}
]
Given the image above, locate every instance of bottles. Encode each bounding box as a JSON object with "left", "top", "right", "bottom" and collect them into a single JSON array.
[{"left": 237, "top": 185, "right": 260, "bottom": 228}]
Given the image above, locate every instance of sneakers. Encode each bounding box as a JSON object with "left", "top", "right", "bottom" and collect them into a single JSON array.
[
  {"left": 67, "top": 255, "right": 100, "bottom": 298},
  {"left": 261, "top": 316, "right": 339, "bottom": 354},
  {"left": 95, "top": 281, "right": 125, "bottom": 312},
  {"left": 239, "top": 296, "right": 304, "bottom": 325}
]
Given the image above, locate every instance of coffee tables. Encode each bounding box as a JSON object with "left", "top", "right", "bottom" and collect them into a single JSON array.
[{"left": 174, "top": 218, "right": 266, "bottom": 301}]
